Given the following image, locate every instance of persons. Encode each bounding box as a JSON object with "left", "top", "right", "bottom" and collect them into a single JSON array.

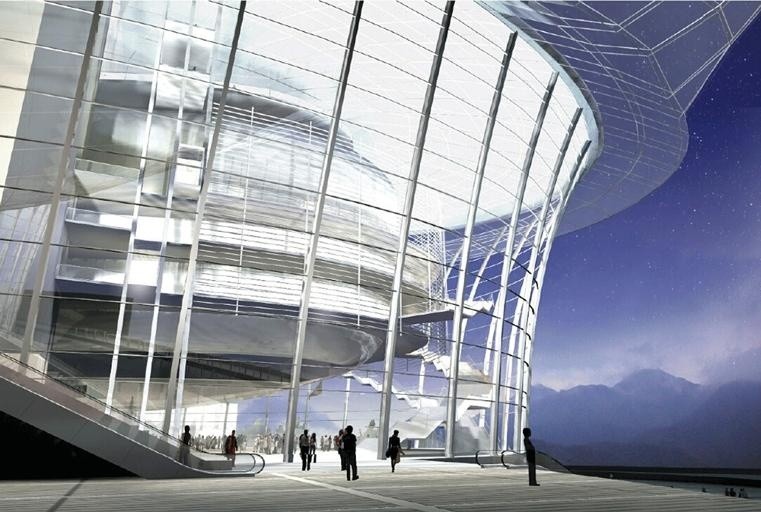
[
  {"left": 178, "top": 425, "right": 359, "bottom": 481},
  {"left": 389, "top": 430, "right": 405, "bottom": 472},
  {"left": 724, "top": 486, "right": 748, "bottom": 499},
  {"left": 523, "top": 427, "right": 541, "bottom": 486}
]
